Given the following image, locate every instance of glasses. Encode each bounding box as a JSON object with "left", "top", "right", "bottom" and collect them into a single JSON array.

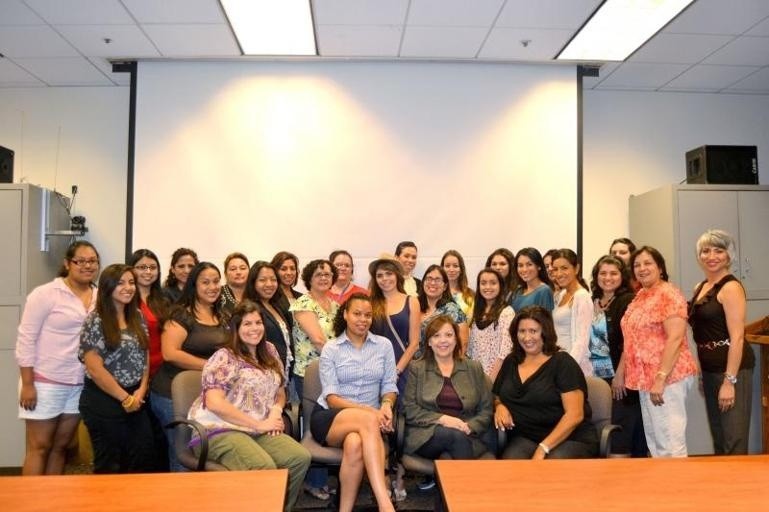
[
  {"left": 66, "top": 257, "right": 99, "bottom": 264},
  {"left": 313, "top": 271, "right": 334, "bottom": 278},
  {"left": 134, "top": 265, "right": 157, "bottom": 270}
]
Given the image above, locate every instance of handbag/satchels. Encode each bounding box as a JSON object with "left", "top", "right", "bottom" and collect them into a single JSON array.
[{"left": 414, "top": 346, "right": 426, "bottom": 359}]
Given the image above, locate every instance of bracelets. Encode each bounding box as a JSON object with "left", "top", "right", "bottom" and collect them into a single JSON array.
[
  {"left": 381, "top": 398, "right": 394, "bottom": 408},
  {"left": 723, "top": 371, "right": 738, "bottom": 384},
  {"left": 122, "top": 394, "right": 134, "bottom": 408},
  {"left": 538, "top": 442, "right": 550, "bottom": 455},
  {"left": 396, "top": 367, "right": 402, "bottom": 376},
  {"left": 270, "top": 405, "right": 283, "bottom": 413},
  {"left": 656, "top": 370, "right": 667, "bottom": 377}
]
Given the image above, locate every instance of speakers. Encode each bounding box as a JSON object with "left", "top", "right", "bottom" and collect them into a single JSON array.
[{"left": 685, "top": 145, "right": 759, "bottom": 183}]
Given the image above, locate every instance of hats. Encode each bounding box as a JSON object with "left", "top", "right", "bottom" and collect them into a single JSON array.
[{"left": 367, "top": 252, "right": 406, "bottom": 277}]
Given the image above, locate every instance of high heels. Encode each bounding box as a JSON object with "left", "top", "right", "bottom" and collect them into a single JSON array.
[{"left": 391, "top": 480, "right": 407, "bottom": 502}]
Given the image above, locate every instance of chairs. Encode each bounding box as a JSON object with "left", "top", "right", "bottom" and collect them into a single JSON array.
[
  {"left": 397, "top": 413, "right": 496, "bottom": 494},
  {"left": 496, "top": 376, "right": 623, "bottom": 458},
  {"left": 300, "top": 363, "right": 396, "bottom": 512},
  {"left": 161, "top": 371, "right": 298, "bottom": 470}
]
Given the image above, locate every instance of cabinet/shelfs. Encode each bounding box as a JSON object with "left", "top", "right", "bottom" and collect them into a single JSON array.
[
  {"left": 0, "top": 184, "right": 70, "bottom": 467},
  {"left": 627, "top": 185, "right": 769, "bottom": 325}
]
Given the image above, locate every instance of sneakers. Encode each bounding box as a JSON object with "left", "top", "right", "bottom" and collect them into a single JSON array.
[
  {"left": 415, "top": 475, "right": 436, "bottom": 489},
  {"left": 303, "top": 482, "right": 330, "bottom": 500}
]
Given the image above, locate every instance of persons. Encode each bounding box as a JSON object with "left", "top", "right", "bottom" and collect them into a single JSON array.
[
  {"left": 463, "top": 268, "right": 515, "bottom": 385},
  {"left": 587, "top": 254, "right": 649, "bottom": 458},
  {"left": 187, "top": 299, "right": 312, "bottom": 512},
  {"left": 491, "top": 305, "right": 599, "bottom": 459},
  {"left": 484, "top": 247, "right": 524, "bottom": 307},
  {"left": 160, "top": 248, "right": 200, "bottom": 313},
  {"left": 543, "top": 249, "right": 561, "bottom": 291},
  {"left": 287, "top": 259, "right": 341, "bottom": 500},
  {"left": 324, "top": 250, "right": 371, "bottom": 304},
  {"left": 363, "top": 252, "right": 421, "bottom": 400},
  {"left": 75, "top": 262, "right": 161, "bottom": 474},
  {"left": 270, "top": 250, "right": 303, "bottom": 330},
  {"left": 608, "top": 238, "right": 643, "bottom": 295},
  {"left": 506, "top": 247, "right": 555, "bottom": 311},
  {"left": 411, "top": 263, "right": 469, "bottom": 490},
  {"left": 128, "top": 249, "right": 163, "bottom": 438},
  {"left": 214, "top": 252, "right": 250, "bottom": 320},
  {"left": 14, "top": 240, "right": 102, "bottom": 475},
  {"left": 309, "top": 292, "right": 400, "bottom": 512},
  {"left": 619, "top": 245, "right": 699, "bottom": 458},
  {"left": 439, "top": 249, "right": 476, "bottom": 326},
  {"left": 150, "top": 261, "right": 233, "bottom": 471},
  {"left": 401, "top": 315, "right": 494, "bottom": 459},
  {"left": 549, "top": 248, "right": 594, "bottom": 378},
  {"left": 688, "top": 227, "right": 756, "bottom": 454},
  {"left": 238, "top": 259, "right": 295, "bottom": 388},
  {"left": 394, "top": 241, "right": 421, "bottom": 297}
]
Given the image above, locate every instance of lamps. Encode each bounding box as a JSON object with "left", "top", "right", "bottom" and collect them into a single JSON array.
[
  {"left": 553, "top": 0, "right": 696, "bottom": 62},
  {"left": 215, "top": 0, "right": 319, "bottom": 56}
]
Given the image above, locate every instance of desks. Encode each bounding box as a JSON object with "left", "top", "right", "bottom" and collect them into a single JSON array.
[
  {"left": 436, "top": 455, "right": 769, "bottom": 511},
  {"left": 0, "top": 468, "right": 289, "bottom": 511}
]
